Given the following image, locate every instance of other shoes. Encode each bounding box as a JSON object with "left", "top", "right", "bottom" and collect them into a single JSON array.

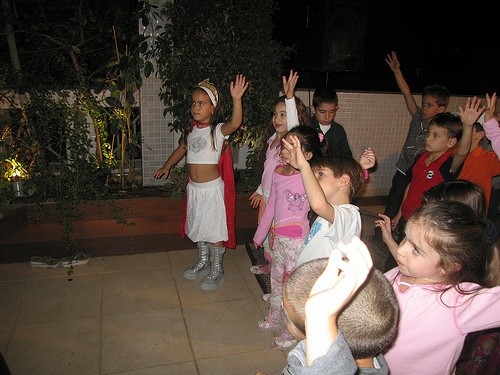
[
  {"left": 257, "top": 320, "right": 273, "bottom": 331},
  {"left": 61, "top": 252, "right": 91, "bottom": 268},
  {"left": 30, "top": 256, "right": 60, "bottom": 268},
  {"left": 263, "top": 293, "right": 271, "bottom": 303},
  {"left": 272, "top": 336, "right": 298, "bottom": 350},
  {"left": 250, "top": 265, "right": 271, "bottom": 274}
]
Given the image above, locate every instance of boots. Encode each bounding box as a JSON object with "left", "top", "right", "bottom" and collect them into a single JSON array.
[
  {"left": 201, "top": 245, "right": 225, "bottom": 291},
  {"left": 184, "top": 242, "right": 211, "bottom": 280}
]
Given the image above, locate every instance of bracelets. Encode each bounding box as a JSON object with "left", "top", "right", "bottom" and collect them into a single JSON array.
[{"left": 285, "top": 94, "right": 294, "bottom": 100}]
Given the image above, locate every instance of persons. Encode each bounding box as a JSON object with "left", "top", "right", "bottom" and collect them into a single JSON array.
[
  {"left": 153, "top": 74, "right": 250, "bottom": 283},
  {"left": 381, "top": 199, "right": 500, "bottom": 375},
  {"left": 281, "top": 136, "right": 362, "bottom": 268},
  {"left": 374, "top": 179, "right": 500, "bottom": 375},
  {"left": 253, "top": 129, "right": 325, "bottom": 347},
  {"left": 250, "top": 69, "right": 312, "bottom": 300},
  {"left": 282, "top": 236, "right": 400, "bottom": 375},
  {"left": 385, "top": 50, "right": 500, "bottom": 274},
  {"left": 304, "top": 85, "right": 377, "bottom": 171}
]
